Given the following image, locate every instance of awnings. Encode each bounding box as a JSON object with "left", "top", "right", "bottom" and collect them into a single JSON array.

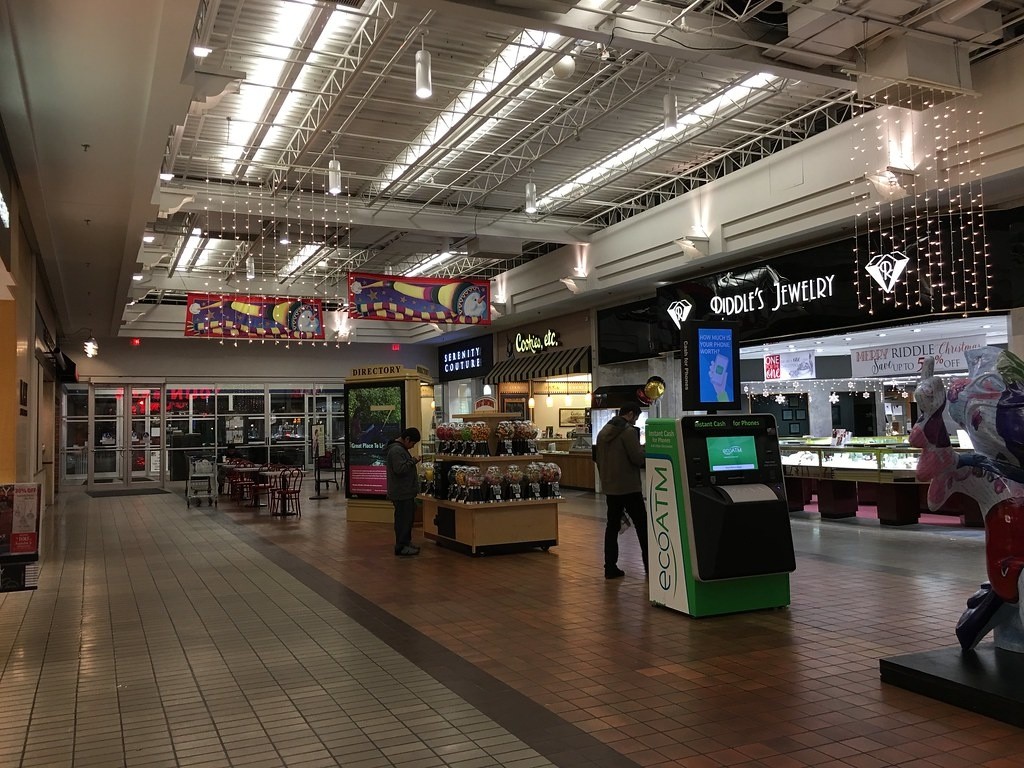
[{"left": 485, "top": 345, "right": 592, "bottom": 384}]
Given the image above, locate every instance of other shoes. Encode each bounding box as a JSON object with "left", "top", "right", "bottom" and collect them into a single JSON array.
[
  {"left": 407, "top": 542, "right": 421, "bottom": 550},
  {"left": 604, "top": 564, "right": 625, "bottom": 579},
  {"left": 395, "top": 546, "right": 420, "bottom": 556},
  {"left": 644, "top": 566, "right": 649, "bottom": 573}
]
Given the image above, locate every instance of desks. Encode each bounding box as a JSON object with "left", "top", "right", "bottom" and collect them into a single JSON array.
[
  {"left": 233, "top": 467, "right": 274, "bottom": 508},
  {"left": 222, "top": 464, "right": 262, "bottom": 501},
  {"left": 260, "top": 471, "right": 310, "bottom": 516}
]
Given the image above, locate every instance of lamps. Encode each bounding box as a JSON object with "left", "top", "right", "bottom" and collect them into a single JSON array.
[
  {"left": 280, "top": 222, "right": 289, "bottom": 245},
  {"left": 160, "top": 173, "right": 175, "bottom": 182},
  {"left": 525, "top": 168, "right": 537, "bottom": 213},
  {"left": 246, "top": 252, "right": 255, "bottom": 278},
  {"left": 57, "top": 327, "right": 98, "bottom": 357},
  {"left": 572, "top": 267, "right": 589, "bottom": 277},
  {"left": 692, "top": 224, "right": 710, "bottom": 236},
  {"left": 193, "top": 42, "right": 214, "bottom": 58},
  {"left": 415, "top": 29, "right": 432, "bottom": 98},
  {"left": 528, "top": 380, "right": 535, "bottom": 408},
  {"left": 546, "top": 381, "right": 553, "bottom": 408},
  {"left": 586, "top": 373, "right": 591, "bottom": 401},
  {"left": 442, "top": 237, "right": 450, "bottom": 258},
  {"left": 329, "top": 143, "right": 341, "bottom": 195},
  {"left": 663, "top": 81, "right": 678, "bottom": 133},
  {"left": 565, "top": 374, "right": 571, "bottom": 406},
  {"left": 889, "top": 152, "right": 913, "bottom": 170}
]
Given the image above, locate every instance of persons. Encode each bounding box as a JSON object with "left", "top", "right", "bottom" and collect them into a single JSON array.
[
  {"left": 382, "top": 427, "right": 424, "bottom": 555},
  {"left": 908, "top": 346, "right": 1024, "bottom": 650},
  {"left": 596, "top": 402, "right": 649, "bottom": 579},
  {"left": 313, "top": 429, "right": 320, "bottom": 457}
]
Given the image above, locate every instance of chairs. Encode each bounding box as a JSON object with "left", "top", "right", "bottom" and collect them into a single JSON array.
[
  {"left": 220, "top": 457, "right": 303, "bottom": 521},
  {"left": 336, "top": 445, "right": 345, "bottom": 483}
]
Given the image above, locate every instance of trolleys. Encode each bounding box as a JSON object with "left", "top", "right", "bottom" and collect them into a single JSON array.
[{"left": 184, "top": 451, "right": 218, "bottom": 508}]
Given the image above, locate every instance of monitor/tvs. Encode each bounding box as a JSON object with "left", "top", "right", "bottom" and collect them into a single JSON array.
[{"left": 706, "top": 436, "right": 758, "bottom": 472}]
